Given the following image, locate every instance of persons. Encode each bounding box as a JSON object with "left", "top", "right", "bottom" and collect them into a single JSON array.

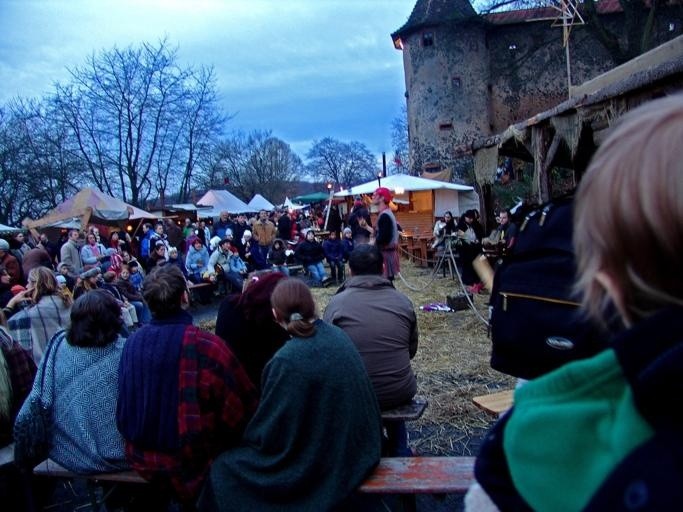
[
  {"left": 433, "top": 205, "right": 516, "bottom": 268},
  {"left": 1, "top": 185, "right": 420, "bottom": 511},
  {"left": 459, "top": 88, "right": 682, "bottom": 511}
]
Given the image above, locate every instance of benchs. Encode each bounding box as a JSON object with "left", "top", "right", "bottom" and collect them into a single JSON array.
[
  {"left": 397, "top": 229, "right": 497, "bottom": 279},
  {"left": 32, "top": 454, "right": 475, "bottom": 511},
  {"left": 189, "top": 258, "right": 347, "bottom": 311},
  {"left": 470, "top": 388, "right": 516, "bottom": 421},
  {"left": 382, "top": 399, "right": 429, "bottom": 457}
]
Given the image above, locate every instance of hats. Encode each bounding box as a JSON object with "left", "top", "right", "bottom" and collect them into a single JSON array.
[
  {"left": 57, "top": 262, "right": 70, "bottom": 273},
  {"left": 79, "top": 265, "right": 101, "bottom": 279},
  {"left": 0, "top": 238, "right": 9, "bottom": 250},
  {"left": 375, "top": 187, "right": 392, "bottom": 203},
  {"left": 343, "top": 227, "right": 353, "bottom": 235},
  {"left": 219, "top": 237, "right": 230, "bottom": 247},
  {"left": 303, "top": 227, "right": 316, "bottom": 239}
]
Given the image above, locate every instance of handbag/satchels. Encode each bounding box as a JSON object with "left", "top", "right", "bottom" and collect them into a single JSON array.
[{"left": 13, "top": 396, "right": 56, "bottom": 467}]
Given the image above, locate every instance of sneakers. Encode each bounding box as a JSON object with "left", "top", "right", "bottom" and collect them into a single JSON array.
[{"left": 321, "top": 276, "right": 333, "bottom": 288}]
